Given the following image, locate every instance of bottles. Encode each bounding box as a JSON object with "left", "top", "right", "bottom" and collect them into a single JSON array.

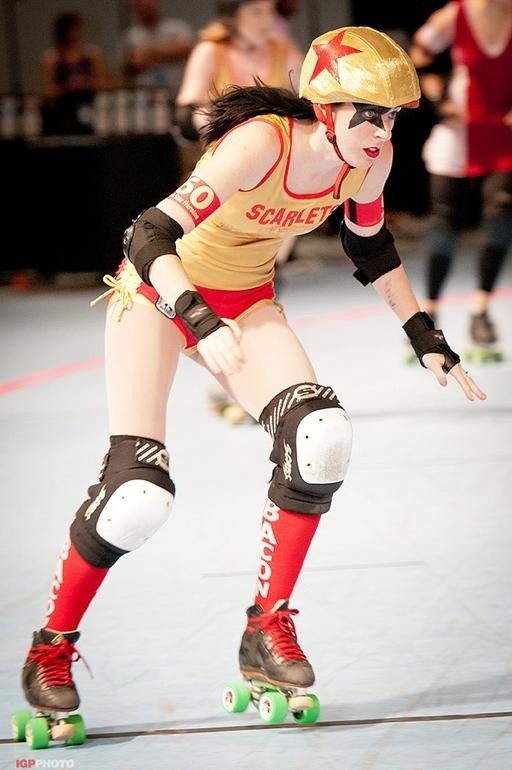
[
  {"left": 0, "top": 96, "right": 42, "bottom": 141},
  {"left": 93, "top": 86, "right": 171, "bottom": 138}
]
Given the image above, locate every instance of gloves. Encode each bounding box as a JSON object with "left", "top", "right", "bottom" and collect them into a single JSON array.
[
  {"left": 175, "top": 290, "right": 236, "bottom": 345},
  {"left": 402, "top": 310, "right": 461, "bottom": 374}
]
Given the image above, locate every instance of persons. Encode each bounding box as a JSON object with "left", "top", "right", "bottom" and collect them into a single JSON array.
[{"left": 0, "top": 1, "right": 512, "bottom": 712}]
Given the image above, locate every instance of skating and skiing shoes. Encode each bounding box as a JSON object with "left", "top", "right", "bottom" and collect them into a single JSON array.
[
  {"left": 220, "top": 599, "right": 321, "bottom": 727},
  {"left": 10, "top": 628, "right": 96, "bottom": 751},
  {"left": 465, "top": 312, "right": 504, "bottom": 366}
]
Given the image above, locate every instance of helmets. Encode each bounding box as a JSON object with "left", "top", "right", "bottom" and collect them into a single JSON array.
[{"left": 297, "top": 26, "right": 423, "bottom": 110}]
